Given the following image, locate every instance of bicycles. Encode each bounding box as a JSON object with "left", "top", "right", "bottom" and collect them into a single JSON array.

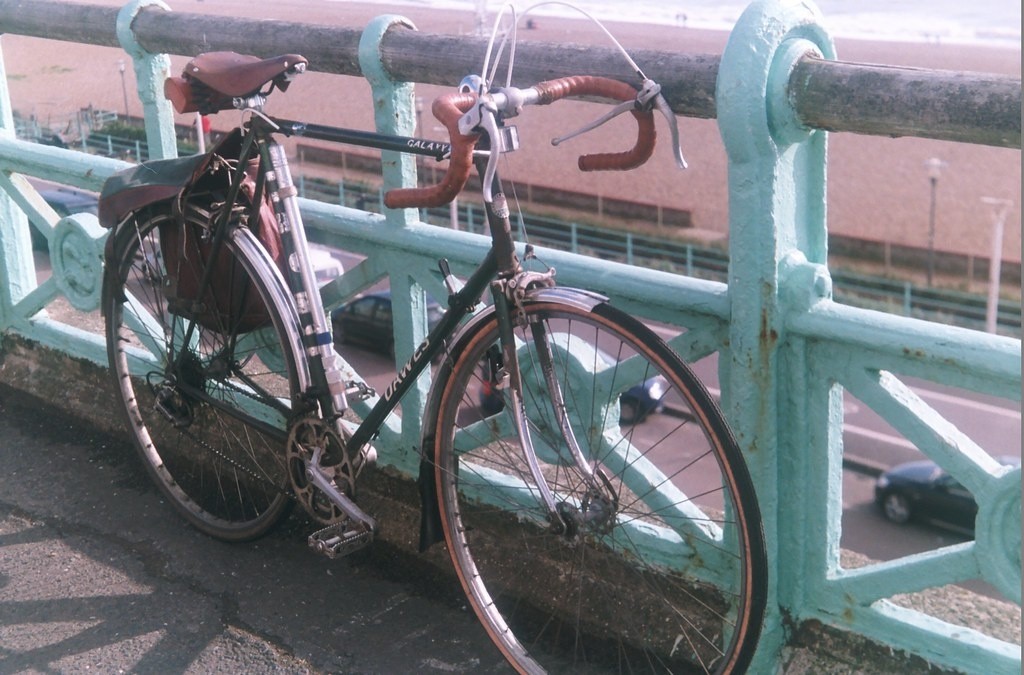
[{"left": 98, "top": 49, "right": 768, "bottom": 675}]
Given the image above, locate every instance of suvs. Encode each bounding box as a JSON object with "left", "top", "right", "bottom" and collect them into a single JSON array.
[{"left": 28, "top": 188, "right": 100, "bottom": 254}]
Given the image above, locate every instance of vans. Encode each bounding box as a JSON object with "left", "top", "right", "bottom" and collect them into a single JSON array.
[{"left": 306, "top": 248, "right": 343, "bottom": 292}]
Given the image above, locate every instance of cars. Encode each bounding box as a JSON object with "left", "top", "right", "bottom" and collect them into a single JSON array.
[
  {"left": 477, "top": 346, "right": 664, "bottom": 426},
  {"left": 330, "top": 288, "right": 445, "bottom": 363},
  {"left": 140, "top": 247, "right": 167, "bottom": 284},
  {"left": 873, "top": 457, "right": 1020, "bottom": 538}
]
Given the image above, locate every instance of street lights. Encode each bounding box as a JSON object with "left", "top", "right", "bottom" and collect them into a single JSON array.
[
  {"left": 925, "top": 158, "right": 942, "bottom": 288},
  {"left": 117, "top": 59, "right": 131, "bottom": 124},
  {"left": 981, "top": 196, "right": 1012, "bottom": 335}
]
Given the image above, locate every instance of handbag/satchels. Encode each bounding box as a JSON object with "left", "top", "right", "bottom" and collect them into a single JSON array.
[{"left": 99, "top": 127, "right": 295, "bottom": 334}]
